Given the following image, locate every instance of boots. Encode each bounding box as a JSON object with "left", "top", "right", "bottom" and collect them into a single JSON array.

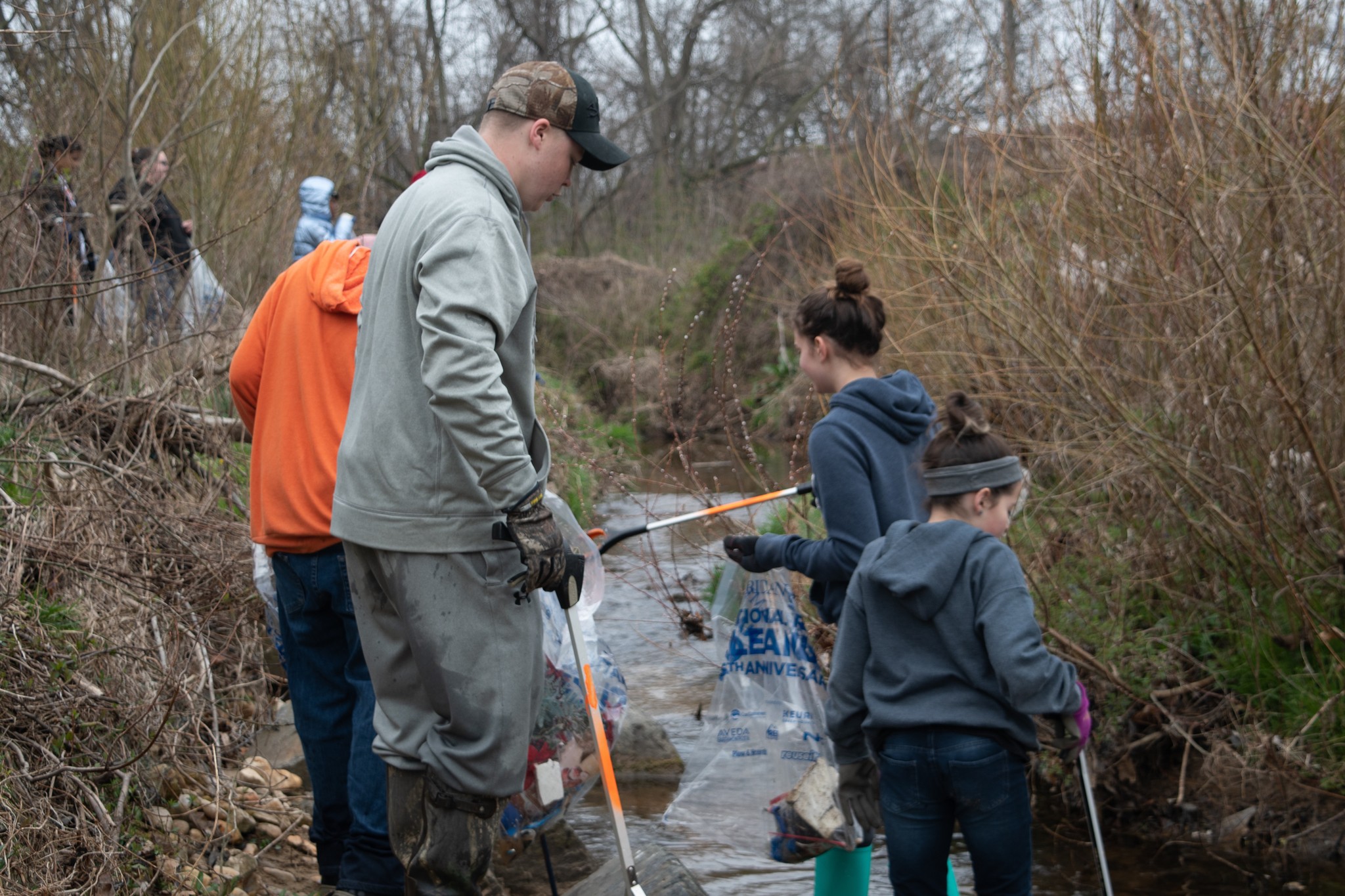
[
  {"left": 386, "top": 760, "right": 428, "bottom": 871},
  {"left": 944, "top": 857, "right": 961, "bottom": 896},
  {"left": 811, "top": 845, "right": 869, "bottom": 896},
  {"left": 402, "top": 773, "right": 514, "bottom": 896}
]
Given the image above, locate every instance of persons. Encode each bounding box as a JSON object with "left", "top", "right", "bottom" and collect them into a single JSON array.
[
  {"left": 230, "top": 233, "right": 405, "bottom": 896},
  {"left": 825, "top": 391, "right": 1090, "bottom": 896},
  {"left": 292, "top": 176, "right": 356, "bottom": 265},
  {"left": 24, "top": 136, "right": 95, "bottom": 320},
  {"left": 328, "top": 61, "right": 632, "bottom": 896},
  {"left": 105, "top": 148, "right": 193, "bottom": 347},
  {"left": 723, "top": 260, "right": 963, "bottom": 896}
]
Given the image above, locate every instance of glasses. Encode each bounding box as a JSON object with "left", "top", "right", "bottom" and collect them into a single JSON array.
[
  {"left": 156, "top": 161, "right": 169, "bottom": 166},
  {"left": 332, "top": 194, "right": 339, "bottom": 199}
]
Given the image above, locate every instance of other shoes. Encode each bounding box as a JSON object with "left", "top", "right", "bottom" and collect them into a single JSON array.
[
  {"left": 332, "top": 886, "right": 379, "bottom": 896},
  {"left": 320, "top": 875, "right": 340, "bottom": 892}
]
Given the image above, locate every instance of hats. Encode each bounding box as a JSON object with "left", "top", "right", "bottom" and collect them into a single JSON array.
[{"left": 486, "top": 61, "right": 632, "bottom": 170}]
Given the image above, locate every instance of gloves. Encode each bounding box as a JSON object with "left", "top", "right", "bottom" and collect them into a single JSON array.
[
  {"left": 724, "top": 531, "right": 795, "bottom": 573},
  {"left": 508, "top": 483, "right": 573, "bottom": 603},
  {"left": 838, "top": 756, "right": 884, "bottom": 832},
  {"left": 1050, "top": 681, "right": 1091, "bottom": 766}
]
